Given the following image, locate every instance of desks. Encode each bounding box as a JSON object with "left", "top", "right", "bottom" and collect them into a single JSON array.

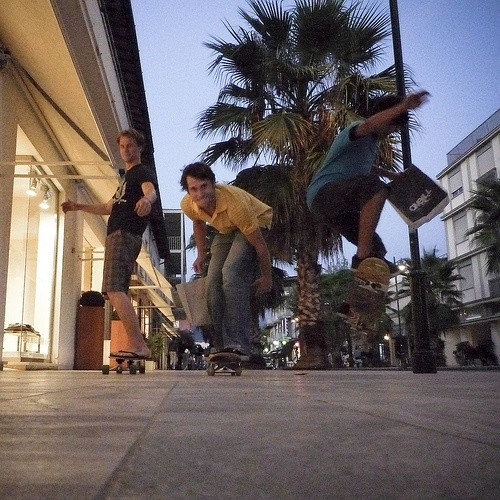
[{"left": 3, "top": 328, "right": 41, "bottom": 353}]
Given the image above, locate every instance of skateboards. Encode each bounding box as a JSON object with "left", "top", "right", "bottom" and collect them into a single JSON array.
[
  {"left": 101, "top": 355, "right": 158, "bottom": 374},
  {"left": 207, "top": 353, "right": 242, "bottom": 378},
  {"left": 341, "top": 258, "right": 390, "bottom": 322}
]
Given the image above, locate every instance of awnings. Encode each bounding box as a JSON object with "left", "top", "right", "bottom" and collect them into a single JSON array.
[
  {"left": 144, "top": 267, "right": 177, "bottom": 307},
  {"left": 142, "top": 281, "right": 181, "bottom": 341},
  {"left": 135, "top": 240, "right": 162, "bottom": 288}
]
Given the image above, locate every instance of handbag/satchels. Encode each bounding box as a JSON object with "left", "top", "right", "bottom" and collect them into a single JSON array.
[
  {"left": 175, "top": 272, "right": 214, "bottom": 327},
  {"left": 387, "top": 163, "right": 449, "bottom": 229}
]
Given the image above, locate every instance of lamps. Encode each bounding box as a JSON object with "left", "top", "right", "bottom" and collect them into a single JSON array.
[
  {"left": 25, "top": 170, "right": 38, "bottom": 196},
  {"left": 39, "top": 185, "right": 50, "bottom": 209}
]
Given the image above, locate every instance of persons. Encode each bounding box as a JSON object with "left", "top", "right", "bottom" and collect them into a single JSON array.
[
  {"left": 180, "top": 162, "right": 272, "bottom": 360},
  {"left": 354, "top": 345, "right": 365, "bottom": 367},
  {"left": 61, "top": 129, "right": 156, "bottom": 359},
  {"left": 306, "top": 91, "right": 430, "bottom": 321}
]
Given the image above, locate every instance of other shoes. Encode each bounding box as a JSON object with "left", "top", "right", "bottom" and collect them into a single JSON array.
[
  {"left": 350, "top": 251, "right": 401, "bottom": 276},
  {"left": 209, "top": 347, "right": 249, "bottom": 360}
]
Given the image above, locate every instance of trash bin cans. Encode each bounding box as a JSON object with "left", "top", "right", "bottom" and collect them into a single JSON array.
[{"left": 75, "top": 305, "right": 105, "bottom": 368}]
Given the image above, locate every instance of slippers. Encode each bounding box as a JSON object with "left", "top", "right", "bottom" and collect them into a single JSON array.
[{"left": 110, "top": 351, "right": 154, "bottom": 360}]
